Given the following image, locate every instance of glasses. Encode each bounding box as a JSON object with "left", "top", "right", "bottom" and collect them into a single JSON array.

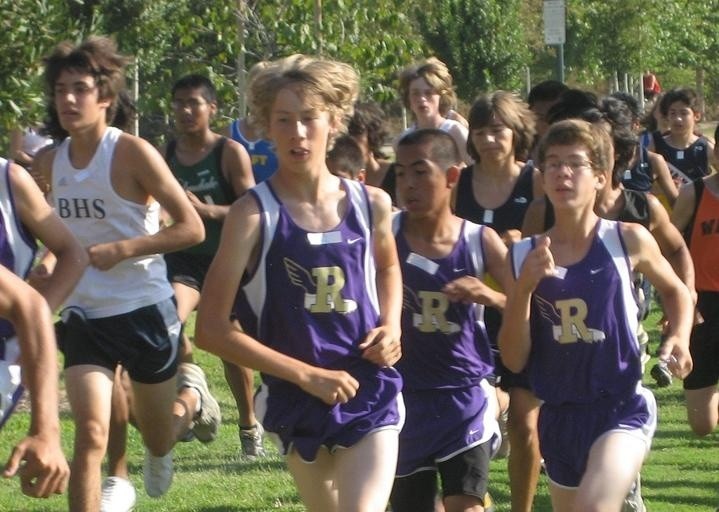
[{"left": 539, "top": 159, "right": 595, "bottom": 175}]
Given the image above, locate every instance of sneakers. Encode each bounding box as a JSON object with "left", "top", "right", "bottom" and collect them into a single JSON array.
[
  {"left": 238, "top": 422, "right": 265, "bottom": 459},
  {"left": 99, "top": 477, "right": 137, "bottom": 512},
  {"left": 623, "top": 475, "right": 646, "bottom": 512},
  {"left": 651, "top": 364, "right": 672, "bottom": 386},
  {"left": 177, "top": 362, "right": 221, "bottom": 443},
  {"left": 497, "top": 409, "right": 511, "bottom": 458},
  {"left": 144, "top": 448, "right": 174, "bottom": 497}
]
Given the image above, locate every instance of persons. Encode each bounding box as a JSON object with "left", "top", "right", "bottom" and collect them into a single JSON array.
[{"left": 2, "top": 24, "right": 719, "bottom": 511}]
